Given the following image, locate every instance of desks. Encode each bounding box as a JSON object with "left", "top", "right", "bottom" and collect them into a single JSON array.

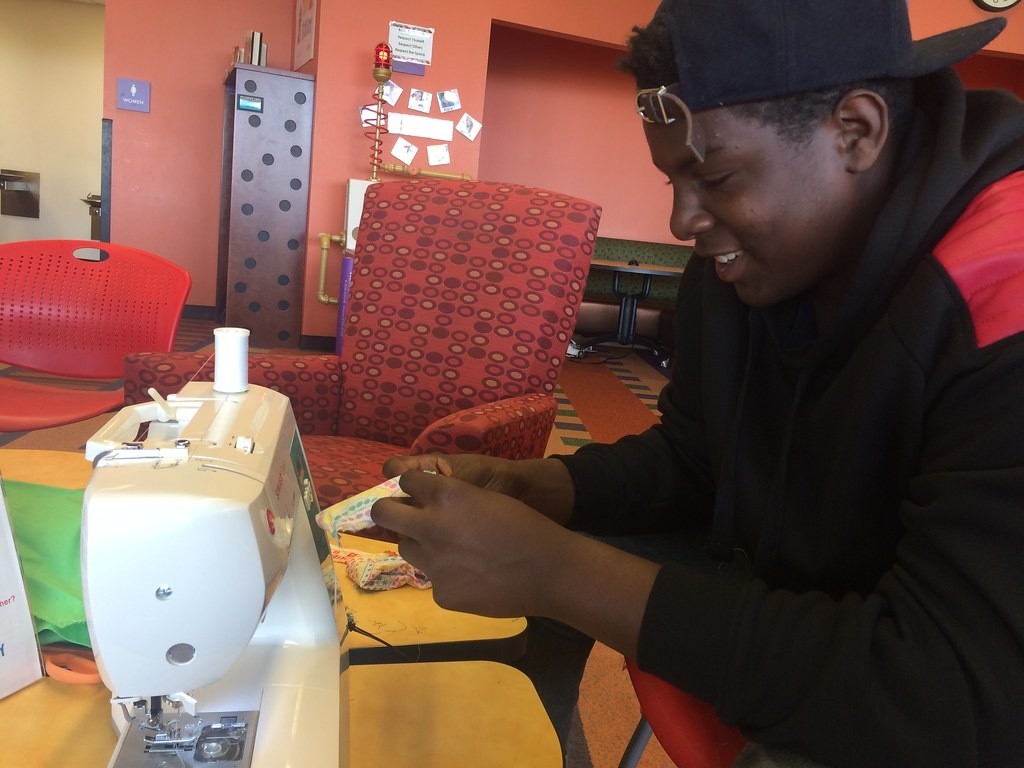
[
  {"left": 579, "top": 256, "right": 685, "bottom": 358},
  {"left": 1, "top": 448, "right": 569, "bottom": 767}
]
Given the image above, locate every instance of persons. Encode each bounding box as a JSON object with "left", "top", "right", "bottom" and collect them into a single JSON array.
[{"left": 369, "top": 0, "right": 1023, "bottom": 768}]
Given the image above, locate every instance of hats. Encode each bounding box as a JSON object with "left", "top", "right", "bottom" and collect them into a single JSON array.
[{"left": 635, "top": 0, "right": 1007, "bottom": 163}]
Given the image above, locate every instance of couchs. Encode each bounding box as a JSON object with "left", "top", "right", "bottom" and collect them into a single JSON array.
[{"left": 121, "top": 174, "right": 602, "bottom": 508}]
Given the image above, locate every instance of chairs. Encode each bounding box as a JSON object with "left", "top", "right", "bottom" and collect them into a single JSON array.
[
  {"left": 562, "top": 641, "right": 745, "bottom": 768},
  {"left": 0, "top": 239, "right": 193, "bottom": 432}
]
